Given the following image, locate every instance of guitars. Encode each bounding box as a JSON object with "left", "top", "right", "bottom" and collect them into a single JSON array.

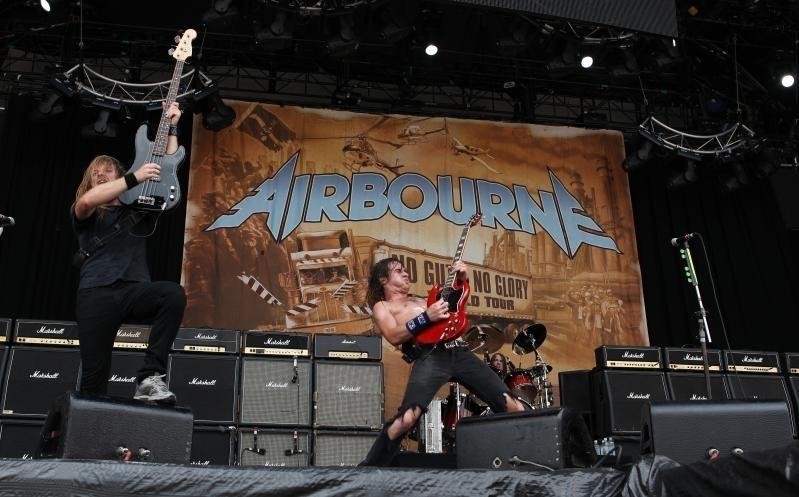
[
  {"left": 415, "top": 214, "right": 484, "bottom": 344},
  {"left": 118, "top": 28, "right": 197, "bottom": 212}
]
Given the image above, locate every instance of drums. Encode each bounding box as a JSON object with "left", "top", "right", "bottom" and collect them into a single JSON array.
[
  {"left": 502, "top": 369, "right": 537, "bottom": 403},
  {"left": 446, "top": 393, "right": 482, "bottom": 439}
]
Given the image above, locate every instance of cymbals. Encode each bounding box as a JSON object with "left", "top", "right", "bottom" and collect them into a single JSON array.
[
  {"left": 528, "top": 365, "right": 552, "bottom": 377},
  {"left": 465, "top": 325, "right": 503, "bottom": 354},
  {"left": 512, "top": 323, "right": 546, "bottom": 355}
]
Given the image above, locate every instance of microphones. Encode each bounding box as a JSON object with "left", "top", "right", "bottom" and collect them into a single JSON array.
[{"left": 671, "top": 233, "right": 693, "bottom": 246}]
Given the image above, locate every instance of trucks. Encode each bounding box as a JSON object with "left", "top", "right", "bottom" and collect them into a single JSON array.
[{"left": 280, "top": 231, "right": 536, "bottom": 345}]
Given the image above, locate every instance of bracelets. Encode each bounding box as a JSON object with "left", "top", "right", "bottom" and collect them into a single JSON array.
[
  {"left": 124, "top": 172, "right": 139, "bottom": 190},
  {"left": 405, "top": 307, "right": 434, "bottom": 339}
]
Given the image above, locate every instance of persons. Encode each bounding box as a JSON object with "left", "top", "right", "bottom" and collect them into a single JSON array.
[
  {"left": 65, "top": 99, "right": 196, "bottom": 409},
  {"left": 366, "top": 255, "right": 526, "bottom": 466},
  {"left": 489, "top": 352, "right": 507, "bottom": 377}
]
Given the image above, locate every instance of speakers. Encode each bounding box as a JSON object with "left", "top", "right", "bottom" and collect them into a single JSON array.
[
  {"left": 561, "top": 345, "right": 798, "bottom": 466},
  {"left": 0, "top": 318, "right": 382, "bottom": 467},
  {"left": 455, "top": 408, "right": 597, "bottom": 469}
]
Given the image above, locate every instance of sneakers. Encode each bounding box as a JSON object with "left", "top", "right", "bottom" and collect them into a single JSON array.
[{"left": 133, "top": 374, "right": 176, "bottom": 406}]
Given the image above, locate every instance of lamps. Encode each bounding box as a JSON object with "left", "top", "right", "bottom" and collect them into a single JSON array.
[
  {"left": 617, "top": 114, "right": 786, "bottom": 192},
  {"left": 54, "top": 59, "right": 238, "bottom": 135},
  {"left": 198, "top": 0, "right": 416, "bottom": 111}
]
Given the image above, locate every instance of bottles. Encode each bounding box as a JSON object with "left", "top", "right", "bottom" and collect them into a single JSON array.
[
  {"left": 593, "top": 439, "right": 599, "bottom": 455},
  {"left": 601, "top": 436, "right": 618, "bottom": 456}
]
